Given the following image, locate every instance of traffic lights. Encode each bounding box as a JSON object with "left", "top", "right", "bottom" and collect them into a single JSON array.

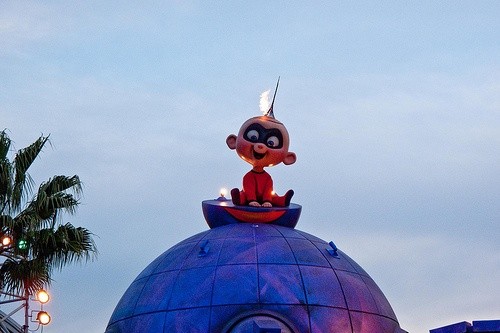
[
  {"left": 1, "top": 235, "right": 31, "bottom": 255},
  {"left": 36, "top": 289, "right": 52, "bottom": 327}
]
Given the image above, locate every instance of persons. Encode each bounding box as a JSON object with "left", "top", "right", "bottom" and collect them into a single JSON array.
[{"left": 226, "top": 116, "right": 296, "bottom": 208}]
